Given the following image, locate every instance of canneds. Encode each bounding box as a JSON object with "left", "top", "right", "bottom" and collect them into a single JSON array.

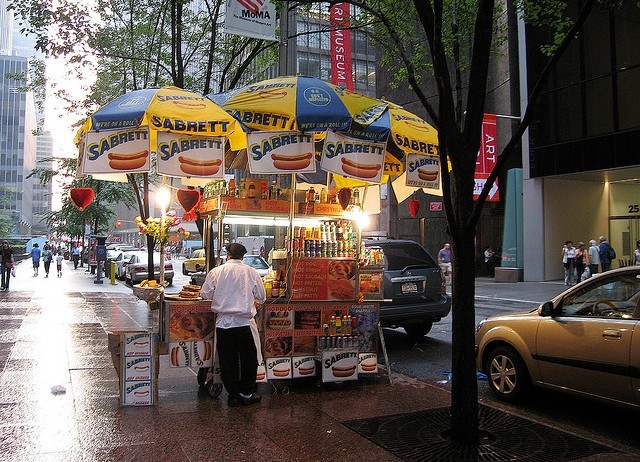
[
  {"left": 321, "top": 241, "right": 327, "bottom": 251},
  {"left": 322, "top": 251, "right": 327, "bottom": 257},
  {"left": 327, "top": 241, "right": 332, "bottom": 251},
  {"left": 299, "top": 239, "right": 305, "bottom": 250},
  {"left": 285, "top": 236, "right": 290, "bottom": 250},
  {"left": 300, "top": 227, "right": 306, "bottom": 238},
  {"left": 294, "top": 239, "right": 299, "bottom": 250},
  {"left": 311, "top": 250, "right": 316, "bottom": 257},
  {"left": 332, "top": 251, "right": 337, "bottom": 257},
  {"left": 327, "top": 251, "right": 332, "bottom": 257},
  {"left": 294, "top": 251, "right": 299, "bottom": 257},
  {"left": 300, "top": 250, "right": 305, "bottom": 258},
  {"left": 306, "top": 227, "right": 312, "bottom": 240},
  {"left": 305, "top": 240, "right": 310, "bottom": 250},
  {"left": 332, "top": 242, "right": 338, "bottom": 251},
  {"left": 316, "top": 240, "right": 321, "bottom": 249},
  {"left": 294, "top": 226, "right": 300, "bottom": 238},
  {"left": 311, "top": 240, "right": 315, "bottom": 250},
  {"left": 317, "top": 250, "right": 321, "bottom": 257},
  {"left": 305, "top": 250, "right": 310, "bottom": 257},
  {"left": 314, "top": 228, "right": 320, "bottom": 238}
]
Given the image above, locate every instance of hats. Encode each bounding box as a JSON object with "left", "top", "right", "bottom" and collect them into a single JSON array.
[{"left": 225, "top": 243, "right": 247, "bottom": 258}]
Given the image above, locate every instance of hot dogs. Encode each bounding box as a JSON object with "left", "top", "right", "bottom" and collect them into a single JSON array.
[
  {"left": 256, "top": 372, "right": 266, "bottom": 380},
  {"left": 405, "top": 121, "right": 429, "bottom": 132},
  {"left": 179, "top": 157, "right": 221, "bottom": 175},
  {"left": 135, "top": 367, "right": 149, "bottom": 371},
  {"left": 271, "top": 152, "right": 313, "bottom": 170},
  {"left": 361, "top": 363, "right": 377, "bottom": 371},
  {"left": 269, "top": 318, "right": 291, "bottom": 330},
  {"left": 273, "top": 369, "right": 290, "bottom": 376},
  {"left": 171, "top": 346, "right": 180, "bottom": 367},
  {"left": 342, "top": 157, "right": 381, "bottom": 178},
  {"left": 135, "top": 391, "right": 150, "bottom": 397},
  {"left": 332, "top": 366, "right": 355, "bottom": 377},
  {"left": 108, "top": 150, "right": 147, "bottom": 169},
  {"left": 174, "top": 101, "right": 206, "bottom": 109},
  {"left": 259, "top": 91, "right": 287, "bottom": 99},
  {"left": 202, "top": 340, "right": 211, "bottom": 361},
  {"left": 418, "top": 169, "right": 438, "bottom": 181},
  {"left": 298, "top": 366, "right": 315, "bottom": 374},
  {"left": 134, "top": 342, "right": 148, "bottom": 347}
]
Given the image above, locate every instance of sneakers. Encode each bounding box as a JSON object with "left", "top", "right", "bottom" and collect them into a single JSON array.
[{"left": 228, "top": 392, "right": 262, "bottom": 407}]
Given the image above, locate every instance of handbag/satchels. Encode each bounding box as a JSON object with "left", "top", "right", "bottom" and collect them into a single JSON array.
[{"left": 2, "top": 249, "right": 14, "bottom": 269}]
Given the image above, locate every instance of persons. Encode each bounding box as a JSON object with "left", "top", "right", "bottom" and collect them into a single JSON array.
[
  {"left": 0, "top": 241, "right": 15, "bottom": 292},
  {"left": 56, "top": 252, "right": 63, "bottom": 278},
  {"left": 199, "top": 243, "right": 267, "bottom": 406},
  {"left": 153, "top": 242, "right": 192, "bottom": 259},
  {"left": 43, "top": 245, "right": 53, "bottom": 277},
  {"left": 50, "top": 243, "right": 62, "bottom": 256},
  {"left": 71, "top": 243, "right": 80, "bottom": 270},
  {"left": 599, "top": 236, "right": 612, "bottom": 273},
  {"left": 438, "top": 243, "right": 452, "bottom": 287},
  {"left": 587, "top": 240, "right": 601, "bottom": 277},
  {"left": 574, "top": 242, "right": 588, "bottom": 284},
  {"left": 560, "top": 241, "right": 575, "bottom": 286},
  {"left": 43, "top": 241, "right": 51, "bottom": 251},
  {"left": 633, "top": 241, "right": 640, "bottom": 265},
  {"left": 31, "top": 243, "right": 41, "bottom": 276},
  {"left": 484, "top": 245, "right": 495, "bottom": 277}
]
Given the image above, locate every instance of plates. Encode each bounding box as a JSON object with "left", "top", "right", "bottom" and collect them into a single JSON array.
[{"left": 174, "top": 295, "right": 203, "bottom": 301}]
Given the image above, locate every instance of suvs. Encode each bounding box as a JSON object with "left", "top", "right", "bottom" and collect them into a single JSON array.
[{"left": 361, "top": 240, "right": 451, "bottom": 337}]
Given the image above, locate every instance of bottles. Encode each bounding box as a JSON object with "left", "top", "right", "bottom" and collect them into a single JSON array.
[
  {"left": 346, "top": 316, "right": 352, "bottom": 335},
  {"left": 335, "top": 317, "right": 341, "bottom": 335},
  {"left": 352, "top": 316, "right": 358, "bottom": 330},
  {"left": 329, "top": 222, "right": 337, "bottom": 240},
  {"left": 354, "top": 187, "right": 361, "bottom": 203},
  {"left": 227, "top": 177, "right": 236, "bottom": 197},
  {"left": 329, "top": 316, "right": 336, "bottom": 335},
  {"left": 319, "top": 187, "right": 328, "bottom": 204},
  {"left": 313, "top": 192, "right": 321, "bottom": 203},
  {"left": 359, "top": 242, "right": 385, "bottom": 267},
  {"left": 320, "top": 222, "right": 326, "bottom": 239},
  {"left": 273, "top": 280, "right": 278, "bottom": 298},
  {"left": 238, "top": 177, "right": 248, "bottom": 199},
  {"left": 335, "top": 222, "right": 343, "bottom": 240},
  {"left": 312, "top": 225, "right": 321, "bottom": 238},
  {"left": 315, "top": 335, "right": 359, "bottom": 357},
  {"left": 258, "top": 178, "right": 267, "bottom": 200},
  {"left": 267, "top": 180, "right": 280, "bottom": 200},
  {"left": 341, "top": 221, "right": 348, "bottom": 241},
  {"left": 324, "top": 222, "right": 331, "bottom": 241},
  {"left": 299, "top": 226, "right": 306, "bottom": 239},
  {"left": 246, "top": 181, "right": 255, "bottom": 199},
  {"left": 294, "top": 225, "right": 301, "bottom": 238},
  {"left": 341, "top": 316, "right": 347, "bottom": 335},
  {"left": 305, "top": 226, "right": 312, "bottom": 238},
  {"left": 265, "top": 280, "right": 272, "bottom": 298},
  {"left": 219, "top": 178, "right": 226, "bottom": 196},
  {"left": 329, "top": 187, "right": 338, "bottom": 204},
  {"left": 308, "top": 186, "right": 315, "bottom": 203},
  {"left": 278, "top": 281, "right": 287, "bottom": 299},
  {"left": 348, "top": 221, "right": 354, "bottom": 240}
]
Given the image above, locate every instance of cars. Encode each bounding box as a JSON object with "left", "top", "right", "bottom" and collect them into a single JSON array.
[
  {"left": 104, "top": 250, "right": 124, "bottom": 272},
  {"left": 115, "top": 251, "right": 147, "bottom": 281},
  {"left": 191, "top": 255, "right": 274, "bottom": 286},
  {"left": 182, "top": 249, "right": 217, "bottom": 275},
  {"left": 125, "top": 253, "right": 174, "bottom": 287},
  {"left": 475, "top": 265, "right": 640, "bottom": 413}
]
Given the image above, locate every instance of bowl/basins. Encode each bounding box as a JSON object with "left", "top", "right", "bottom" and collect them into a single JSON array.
[{"left": 132, "top": 285, "right": 164, "bottom": 301}]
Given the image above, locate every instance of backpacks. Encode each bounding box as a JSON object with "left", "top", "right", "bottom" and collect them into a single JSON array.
[{"left": 603, "top": 243, "right": 616, "bottom": 260}]
